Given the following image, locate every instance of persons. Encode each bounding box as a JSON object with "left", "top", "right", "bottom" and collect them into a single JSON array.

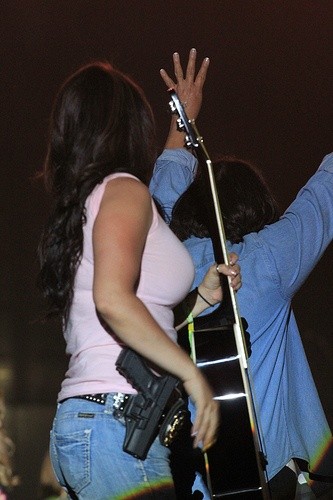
[
  {"left": 169, "top": 142, "right": 333, "bottom": 499},
  {"left": 32, "top": 60, "right": 243, "bottom": 499}
]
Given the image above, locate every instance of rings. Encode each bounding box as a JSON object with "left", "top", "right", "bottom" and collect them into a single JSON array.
[{"left": 216, "top": 264, "right": 220, "bottom": 275}]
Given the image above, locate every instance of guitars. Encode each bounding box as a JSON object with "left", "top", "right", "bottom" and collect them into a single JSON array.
[{"left": 161, "top": 87, "right": 275, "bottom": 498}]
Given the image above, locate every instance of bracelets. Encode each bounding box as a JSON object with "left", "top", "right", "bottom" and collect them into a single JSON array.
[{"left": 194, "top": 286, "right": 217, "bottom": 309}]
